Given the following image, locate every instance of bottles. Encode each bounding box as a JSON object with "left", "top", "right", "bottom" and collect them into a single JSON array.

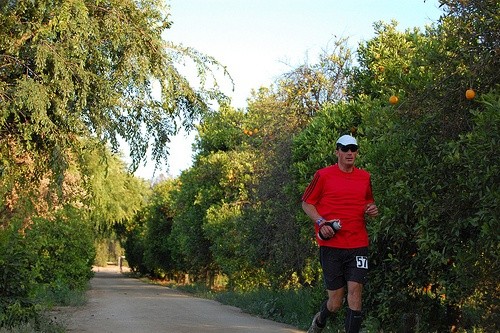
[{"left": 318, "top": 222, "right": 342, "bottom": 240}]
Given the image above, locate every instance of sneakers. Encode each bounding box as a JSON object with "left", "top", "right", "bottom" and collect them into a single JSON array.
[{"left": 306, "top": 312, "right": 325, "bottom": 333}]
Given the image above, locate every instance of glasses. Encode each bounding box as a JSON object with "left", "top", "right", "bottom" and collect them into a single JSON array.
[{"left": 336, "top": 144, "right": 358, "bottom": 152}]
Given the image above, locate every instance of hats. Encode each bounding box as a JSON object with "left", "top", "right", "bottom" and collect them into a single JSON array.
[{"left": 335, "top": 135, "right": 358, "bottom": 149}]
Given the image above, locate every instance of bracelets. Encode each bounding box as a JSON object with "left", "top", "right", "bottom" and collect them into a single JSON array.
[{"left": 317, "top": 218, "right": 324, "bottom": 224}]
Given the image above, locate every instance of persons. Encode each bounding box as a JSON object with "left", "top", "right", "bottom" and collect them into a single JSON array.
[{"left": 302, "top": 135, "right": 382, "bottom": 333}]
